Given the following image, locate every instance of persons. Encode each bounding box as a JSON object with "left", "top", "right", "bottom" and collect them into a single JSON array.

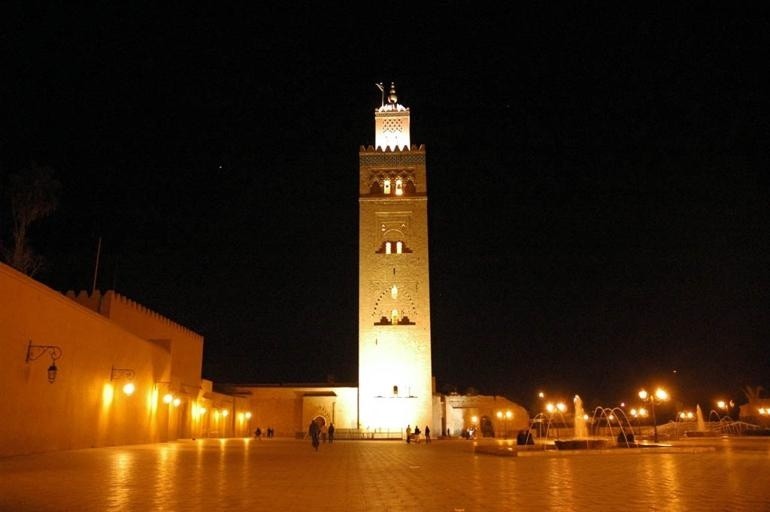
[
  {"left": 415, "top": 426, "right": 420, "bottom": 443},
  {"left": 321, "top": 423, "right": 327, "bottom": 443},
  {"left": 406, "top": 424, "right": 412, "bottom": 444},
  {"left": 252, "top": 426, "right": 274, "bottom": 441},
  {"left": 308, "top": 419, "right": 321, "bottom": 451},
  {"left": 425, "top": 426, "right": 431, "bottom": 443},
  {"left": 328, "top": 422, "right": 335, "bottom": 443}
]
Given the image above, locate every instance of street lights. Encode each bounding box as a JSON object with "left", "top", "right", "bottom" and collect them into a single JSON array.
[
  {"left": 497, "top": 411, "right": 513, "bottom": 440},
  {"left": 546, "top": 402, "right": 566, "bottom": 440},
  {"left": 638, "top": 388, "right": 669, "bottom": 442}
]
